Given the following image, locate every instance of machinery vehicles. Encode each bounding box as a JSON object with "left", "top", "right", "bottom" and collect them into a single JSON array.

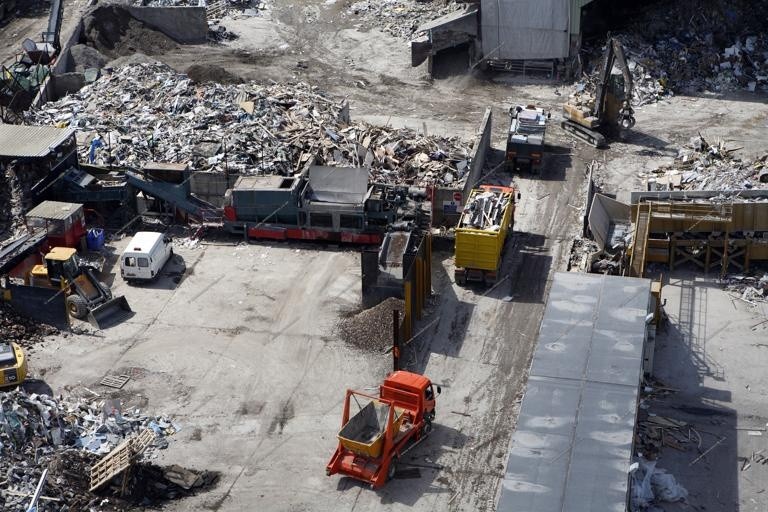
[
  {"left": 0, "top": 341, "right": 29, "bottom": 387},
  {"left": 26, "top": 245, "right": 139, "bottom": 332},
  {"left": 557, "top": 35, "right": 638, "bottom": 154}
]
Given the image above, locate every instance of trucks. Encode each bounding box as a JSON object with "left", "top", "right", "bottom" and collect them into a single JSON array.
[
  {"left": 322, "top": 368, "right": 444, "bottom": 492},
  {"left": 502, "top": 103, "right": 551, "bottom": 174},
  {"left": 451, "top": 183, "right": 523, "bottom": 290}
]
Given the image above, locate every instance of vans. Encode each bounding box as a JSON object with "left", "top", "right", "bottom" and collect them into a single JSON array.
[{"left": 120, "top": 231, "right": 174, "bottom": 282}]
